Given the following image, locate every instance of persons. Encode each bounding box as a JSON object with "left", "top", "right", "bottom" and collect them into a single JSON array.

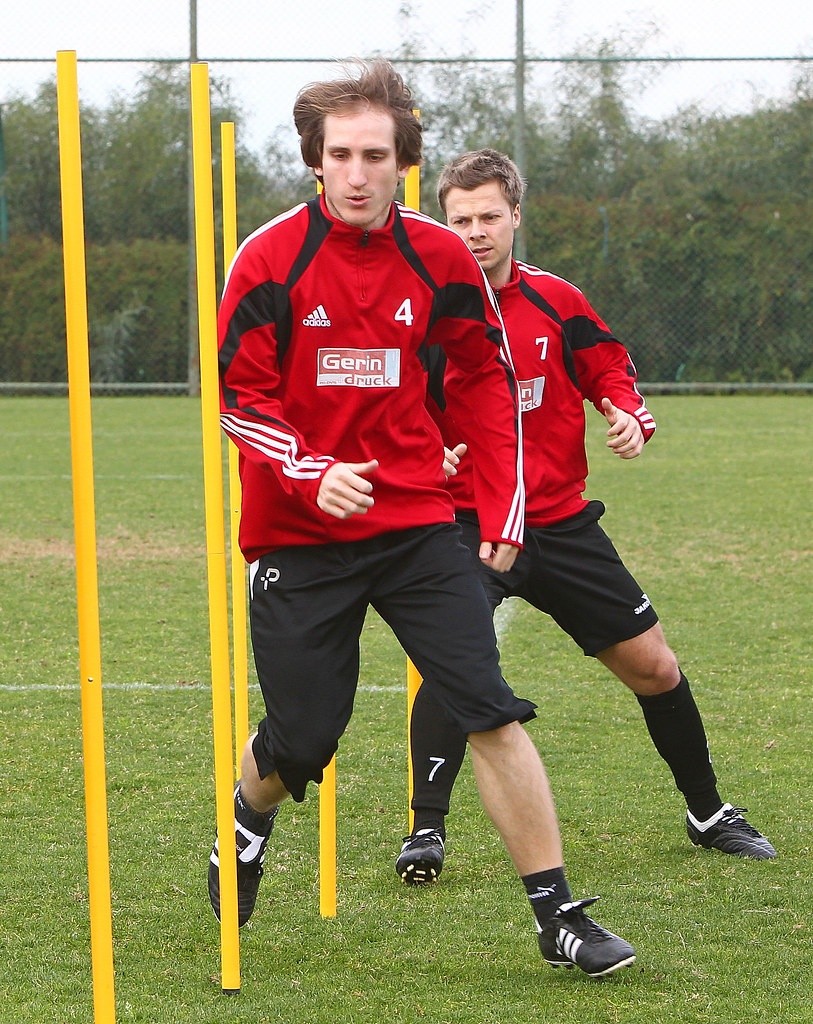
[
  {"left": 394, "top": 150, "right": 777, "bottom": 884},
  {"left": 207, "top": 51, "right": 635, "bottom": 978}
]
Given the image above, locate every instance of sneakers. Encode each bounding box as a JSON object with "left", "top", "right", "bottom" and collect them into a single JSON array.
[
  {"left": 208, "top": 784, "right": 280, "bottom": 929},
  {"left": 686, "top": 803, "right": 777, "bottom": 861},
  {"left": 395, "top": 826, "right": 446, "bottom": 888},
  {"left": 533, "top": 896, "right": 636, "bottom": 981}
]
